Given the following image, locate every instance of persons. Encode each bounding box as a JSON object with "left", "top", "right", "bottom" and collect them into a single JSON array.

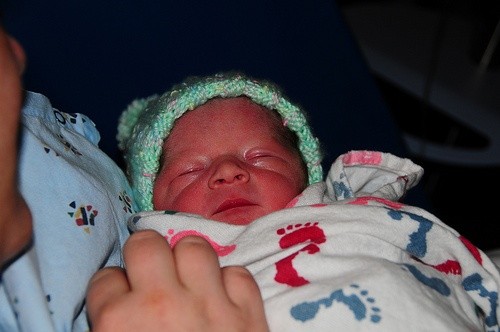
[
  {"left": 117, "top": 75, "right": 500, "bottom": 331},
  {"left": 0, "top": 16, "right": 268, "bottom": 331}
]
